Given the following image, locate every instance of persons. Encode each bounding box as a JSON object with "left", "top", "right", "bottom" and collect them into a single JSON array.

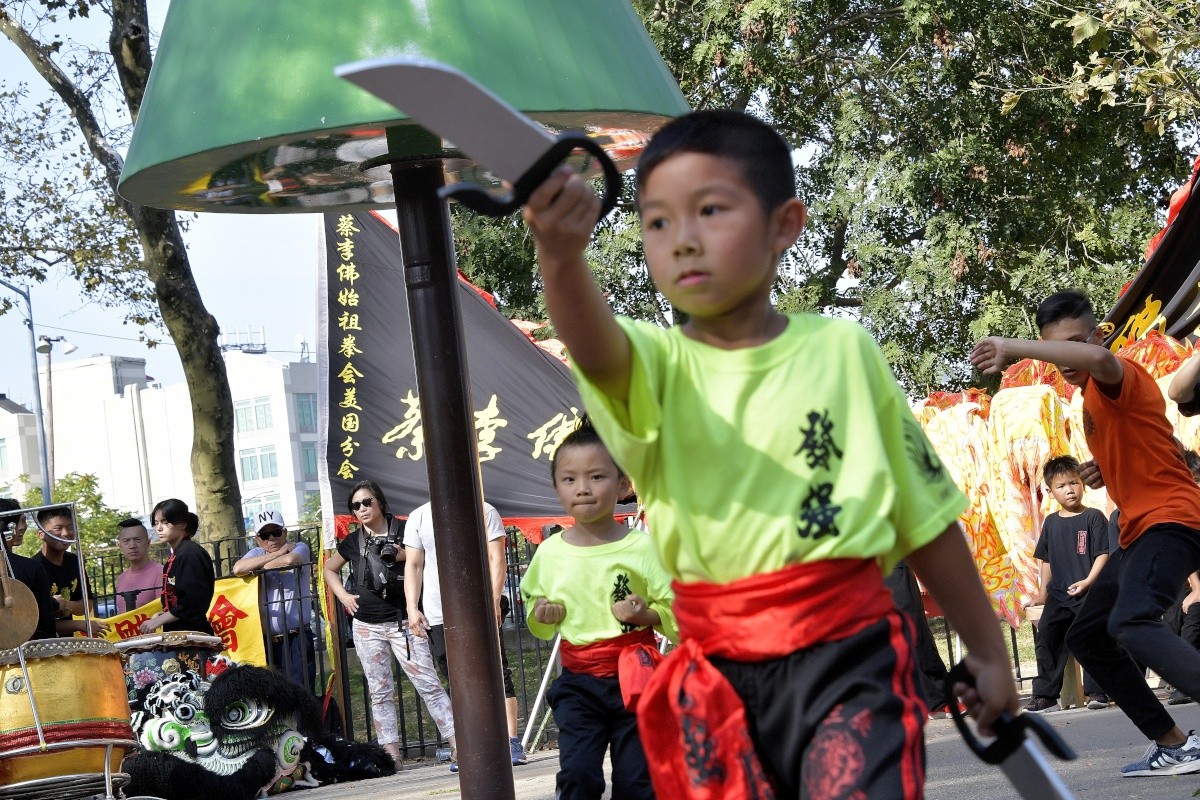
[
  {"left": 502, "top": 102, "right": 1019, "bottom": 800},
  {"left": 1, "top": 497, "right": 73, "bottom": 651},
  {"left": 401, "top": 491, "right": 525, "bottom": 770},
  {"left": 1167, "top": 348, "right": 1200, "bottom": 417},
  {"left": 518, "top": 423, "right": 682, "bottom": 800},
  {"left": 966, "top": 288, "right": 1200, "bottom": 777},
  {"left": 138, "top": 498, "right": 215, "bottom": 635},
  {"left": 232, "top": 509, "right": 317, "bottom": 694},
  {"left": 1160, "top": 571, "right": 1199, "bottom": 704},
  {"left": 26, "top": 504, "right": 112, "bottom": 639},
  {"left": 115, "top": 517, "right": 164, "bottom": 616},
  {"left": 1023, "top": 453, "right": 1111, "bottom": 715},
  {"left": 321, "top": 480, "right": 458, "bottom": 772}
]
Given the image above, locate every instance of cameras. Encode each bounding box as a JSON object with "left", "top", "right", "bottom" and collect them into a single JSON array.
[{"left": 373, "top": 535, "right": 405, "bottom": 568}]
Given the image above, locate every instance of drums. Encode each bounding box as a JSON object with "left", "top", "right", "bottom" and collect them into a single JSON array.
[
  {"left": 114, "top": 631, "right": 223, "bottom": 711},
  {"left": 0, "top": 636, "right": 134, "bottom": 787}
]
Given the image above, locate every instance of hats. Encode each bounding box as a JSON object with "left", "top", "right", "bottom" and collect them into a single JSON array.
[{"left": 254, "top": 510, "right": 284, "bottom": 534}]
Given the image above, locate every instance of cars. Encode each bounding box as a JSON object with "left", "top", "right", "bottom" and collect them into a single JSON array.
[
  {"left": 311, "top": 595, "right": 353, "bottom": 649},
  {"left": 503, "top": 573, "right": 526, "bottom": 614}
]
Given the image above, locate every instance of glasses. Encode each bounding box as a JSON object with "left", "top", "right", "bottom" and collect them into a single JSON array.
[
  {"left": 350, "top": 498, "right": 373, "bottom": 510},
  {"left": 258, "top": 527, "right": 283, "bottom": 541}
]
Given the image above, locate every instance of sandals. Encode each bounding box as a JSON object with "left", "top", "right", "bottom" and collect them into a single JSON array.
[
  {"left": 391, "top": 756, "right": 404, "bottom": 771},
  {"left": 450, "top": 746, "right": 457, "bottom": 763}
]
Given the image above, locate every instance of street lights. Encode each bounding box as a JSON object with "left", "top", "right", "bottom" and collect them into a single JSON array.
[{"left": 35, "top": 334, "right": 79, "bottom": 504}]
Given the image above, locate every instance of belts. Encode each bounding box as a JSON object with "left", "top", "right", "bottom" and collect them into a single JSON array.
[{"left": 271, "top": 625, "right": 310, "bottom": 643}]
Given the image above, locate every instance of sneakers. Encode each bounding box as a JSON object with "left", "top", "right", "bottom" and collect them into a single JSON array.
[
  {"left": 1086, "top": 693, "right": 1110, "bottom": 709},
  {"left": 1021, "top": 696, "right": 1061, "bottom": 714},
  {"left": 450, "top": 763, "right": 458, "bottom": 772},
  {"left": 510, "top": 737, "right": 528, "bottom": 766},
  {"left": 1120, "top": 729, "right": 1200, "bottom": 777}
]
